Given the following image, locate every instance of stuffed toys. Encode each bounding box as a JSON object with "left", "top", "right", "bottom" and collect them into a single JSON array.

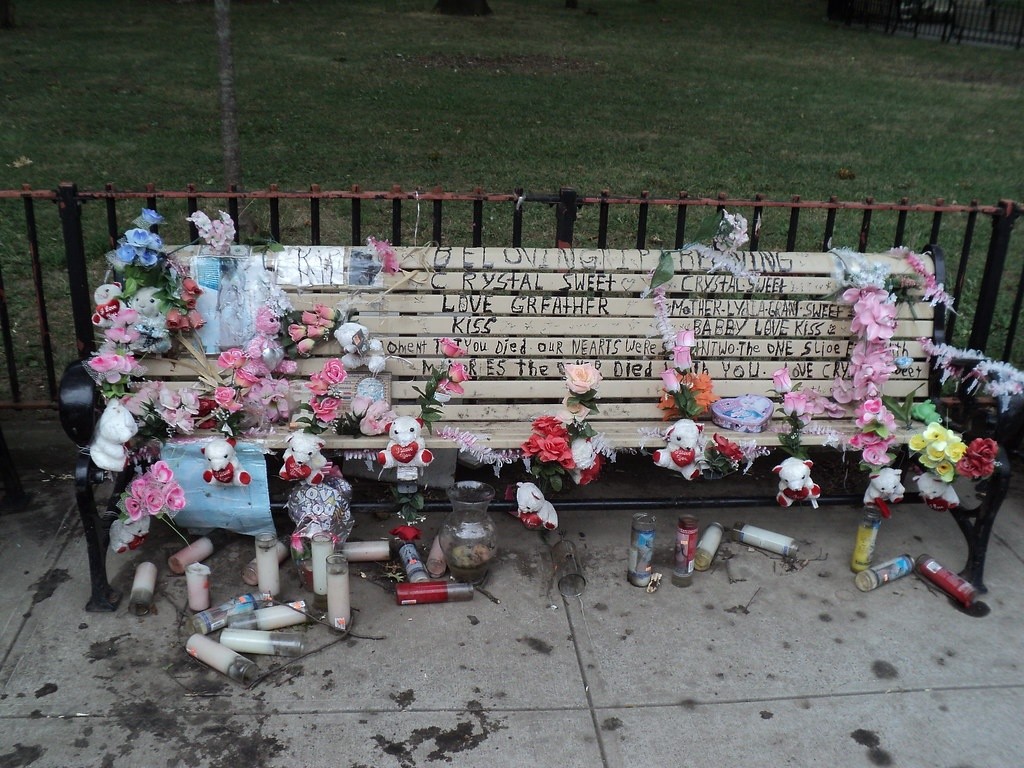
[
  {"left": 652, "top": 418, "right": 705, "bottom": 482},
  {"left": 278, "top": 431, "right": 328, "bottom": 486},
  {"left": 109, "top": 513, "right": 150, "bottom": 553},
  {"left": 862, "top": 467, "right": 906, "bottom": 520},
  {"left": 89, "top": 398, "right": 138, "bottom": 472},
  {"left": 201, "top": 436, "right": 253, "bottom": 486},
  {"left": 244, "top": 305, "right": 297, "bottom": 376},
  {"left": 771, "top": 456, "right": 821, "bottom": 508},
  {"left": 570, "top": 435, "right": 605, "bottom": 485},
  {"left": 91, "top": 281, "right": 173, "bottom": 351},
  {"left": 912, "top": 471, "right": 961, "bottom": 512},
  {"left": 330, "top": 322, "right": 387, "bottom": 372},
  {"left": 514, "top": 481, "right": 559, "bottom": 530},
  {"left": 376, "top": 417, "right": 435, "bottom": 467}
]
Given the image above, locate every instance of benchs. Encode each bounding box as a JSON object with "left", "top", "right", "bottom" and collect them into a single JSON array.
[{"left": 57, "top": 245, "right": 1013, "bottom": 615}]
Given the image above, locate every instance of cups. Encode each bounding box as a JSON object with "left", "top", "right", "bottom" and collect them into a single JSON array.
[{"left": 128, "top": 482, "right": 983, "bottom": 688}]
